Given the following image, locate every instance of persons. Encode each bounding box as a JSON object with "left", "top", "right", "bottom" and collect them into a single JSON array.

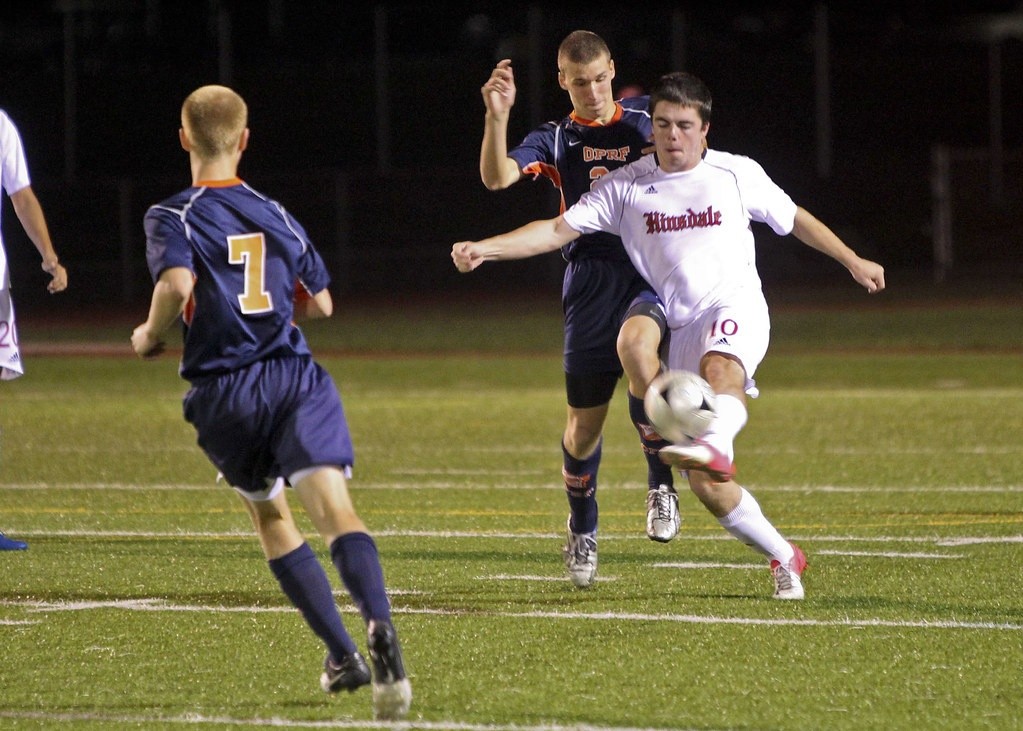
[
  {"left": 0, "top": 109, "right": 68, "bottom": 549},
  {"left": 131, "top": 86, "right": 414, "bottom": 712},
  {"left": 451, "top": 31, "right": 886, "bottom": 599}
]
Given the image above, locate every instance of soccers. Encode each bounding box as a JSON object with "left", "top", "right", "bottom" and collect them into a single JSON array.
[{"left": 645, "top": 370, "right": 713, "bottom": 443}]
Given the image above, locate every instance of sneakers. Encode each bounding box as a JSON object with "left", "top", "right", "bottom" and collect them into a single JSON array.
[
  {"left": 657, "top": 431, "right": 736, "bottom": 482},
  {"left": 367, "top": 618, "right": 412, "bottom": 717},
  {"left": 319, "top": 650, "right": 371, "bottom": 694},
  {"left": 645, "top": 484, "right": 681, "bottom": 543},
  {"left": 770, "top": 542, "right": 809, "bottom": 600},
  {"left": 566, "top": 512, "right": 597, "bottom": 589}
]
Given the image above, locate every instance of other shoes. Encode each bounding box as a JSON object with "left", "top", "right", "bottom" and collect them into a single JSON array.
[{"left": 0, "top": 532, "right": 28, "bottom": 551}]
